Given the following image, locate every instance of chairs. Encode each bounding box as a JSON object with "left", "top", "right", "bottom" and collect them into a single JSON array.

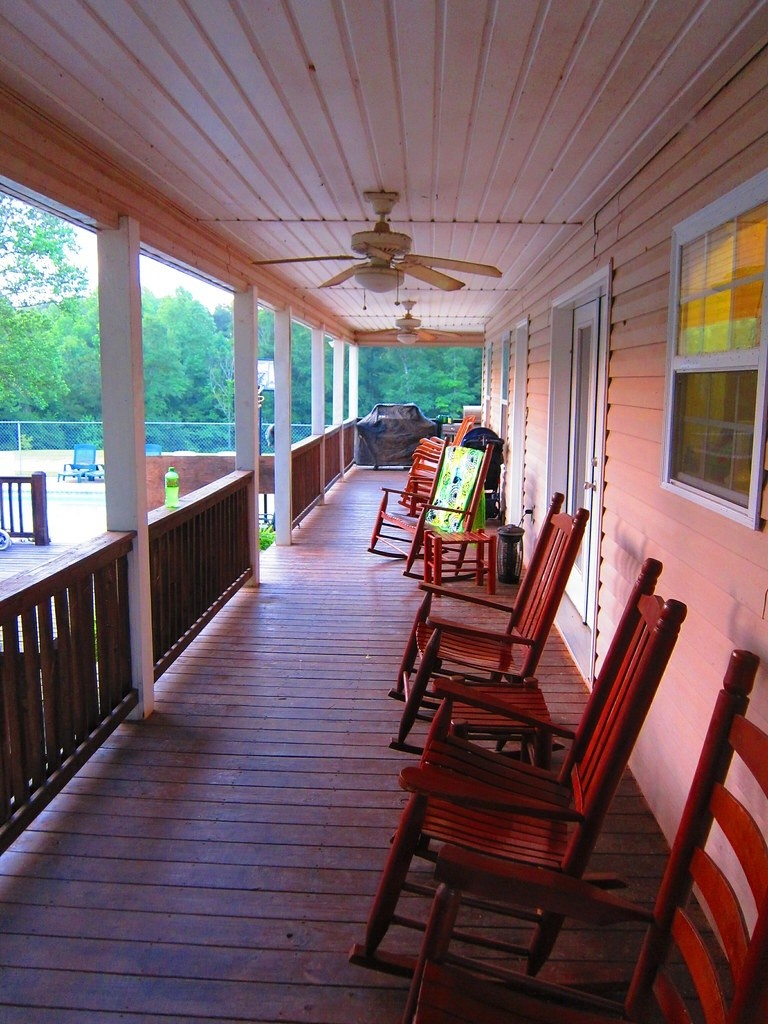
[
  {"left": 350, "top": 558, "right": 685, "bottom": 981},
  {"left": 367, "top": 436, "right": 495, "bottom": 582},
  {"left": 399, "top": 648, "right": 768, "bottom": 1024},
  {"left": 57, "top": 444, "right": 161, "bottom": 482},
  {"left": 388, "top": 492, "right": 589, "bottom": 760},
  {"left": 400, "top": 415, "right": 476, "bottom": 511}
]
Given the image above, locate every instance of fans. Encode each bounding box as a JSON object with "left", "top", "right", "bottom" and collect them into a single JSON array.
[
  {"left": 369, "top": 300, "right": 462, "bottom": 343},
  {"left": 250, "top": 191, "right": 502, "bottom": 293}
]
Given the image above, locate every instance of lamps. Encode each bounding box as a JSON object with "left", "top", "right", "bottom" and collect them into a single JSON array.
[
  {"left": 497, "top": 511, "right": 530, "bottom": 585},
  {"left": 396, "top": 332, "right": 418, "bottom": 345},
  {"left": 354, "top": 266, "right": 402, "bottom": 295}
]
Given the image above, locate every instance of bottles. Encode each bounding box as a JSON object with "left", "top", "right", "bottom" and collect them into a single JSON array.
[
  {"left": 165, "top": 467, "right": 181, "bottom": 510},
  {"left": 438, "top": 414, "right": 454, "bottom": 424}
]
